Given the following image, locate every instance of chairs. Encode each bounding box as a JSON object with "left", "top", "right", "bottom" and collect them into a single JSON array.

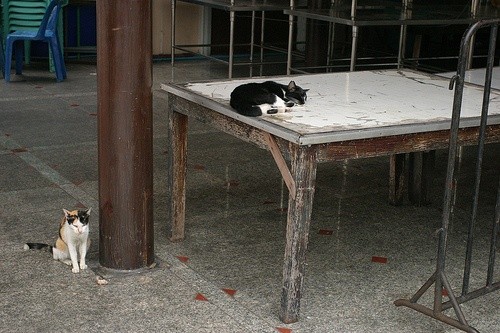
[{"left": 1, "top": 1, "right": 65, "bottom": 84}]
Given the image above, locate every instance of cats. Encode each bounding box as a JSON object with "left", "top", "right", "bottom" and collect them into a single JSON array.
[
  {"left": 229, "top": 80, "right": 311, "bottom": 118},
  {"left": 24, "top": 206, "right": 93, "bottom": 273}
]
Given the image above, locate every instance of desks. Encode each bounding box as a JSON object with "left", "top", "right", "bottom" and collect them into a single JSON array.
[{"left": 160, "top": 0, "right": 500, "bottom": 323}]
